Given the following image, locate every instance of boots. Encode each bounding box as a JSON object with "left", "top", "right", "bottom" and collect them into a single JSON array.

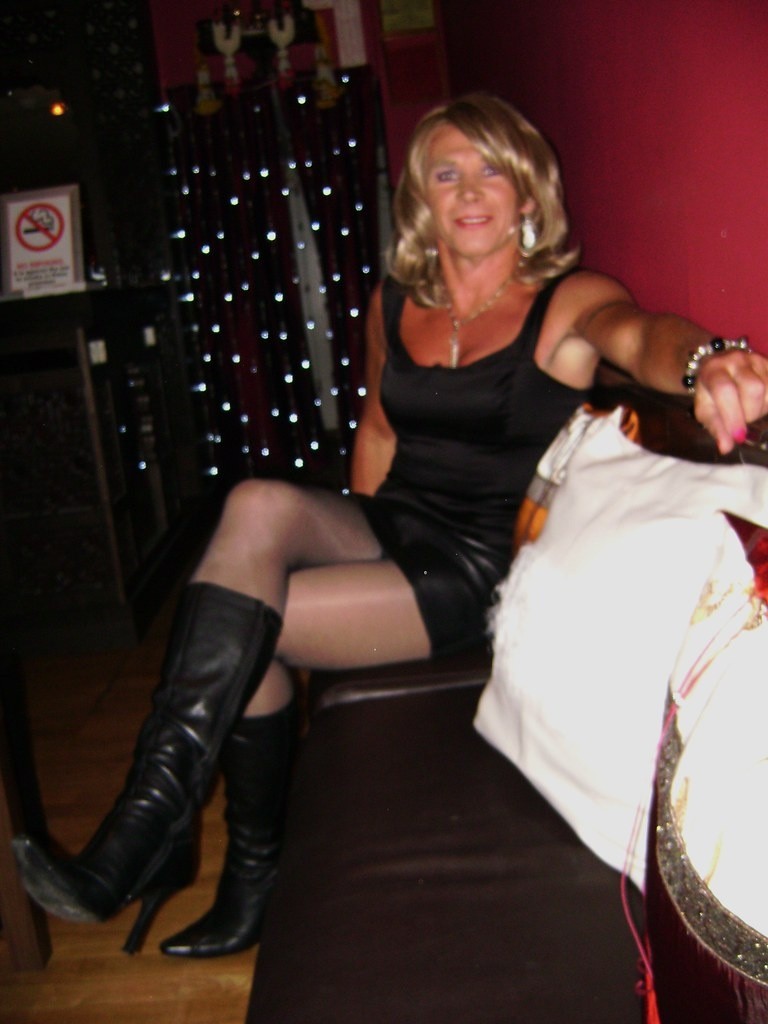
[
  {"left": 12, "top": 575, "right": 283, "bottom": 956},
  {"left": 161, "top": 690, "right": 296, "bottom": 954}
]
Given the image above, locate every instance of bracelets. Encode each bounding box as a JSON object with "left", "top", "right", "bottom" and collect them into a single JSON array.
[{"left": 680, "top": 336, "right": 750, "bottom": 400}]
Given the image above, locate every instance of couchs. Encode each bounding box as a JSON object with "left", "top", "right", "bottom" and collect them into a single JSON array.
[{"left": 249, "top": 383, "right": 768, "bottom": 1024}]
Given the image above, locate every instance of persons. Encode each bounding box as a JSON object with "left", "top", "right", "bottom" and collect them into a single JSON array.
[{"left": 10, "top": 92, "right": 768, "bottom": 958}]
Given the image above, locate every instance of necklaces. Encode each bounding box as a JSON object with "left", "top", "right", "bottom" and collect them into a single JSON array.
[{"left": 431, "top": 263, "right": 524, "bottom": 371}]
